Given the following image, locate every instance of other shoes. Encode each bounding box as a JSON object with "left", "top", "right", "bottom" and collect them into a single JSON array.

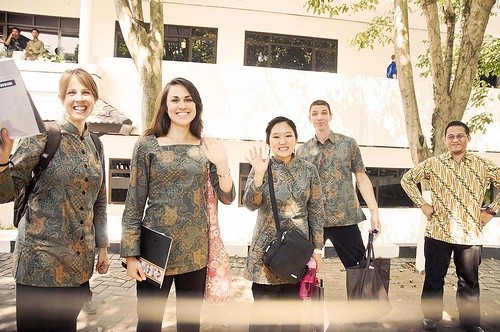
[
  {"left": 422, "top": 316, "right": 438, "bottom": 332},
  {"left": 470, "top": 326, "right": 484, "bottom": 332}
]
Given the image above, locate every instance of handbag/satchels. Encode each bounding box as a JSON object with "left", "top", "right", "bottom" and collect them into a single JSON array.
[
  {"left": 346, "top": 231, "right": 391, "bottom": 301},
  {"left": 205, "top": 237, "right": 232, "bottom": 304},
  {"left": 261, "top": 225, "right": 315, "bottom": 285}
]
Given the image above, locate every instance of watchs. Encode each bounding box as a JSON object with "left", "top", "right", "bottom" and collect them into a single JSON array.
[{"left": 485, "top": 207, "right": 497, "bottom": 216}]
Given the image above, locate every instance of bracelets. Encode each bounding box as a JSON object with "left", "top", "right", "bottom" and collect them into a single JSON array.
[
  {"left": 368, "top": 205, "right": 379, "bottom": 213},
  {"left": 313, "top": 247, "right": 325, "bottom": 258},
  {"left": 215, "top": 168, "right": 232, "bottom": 179},
  {"left": 0, "top": 158, "right": 15, "bottom": 168}
]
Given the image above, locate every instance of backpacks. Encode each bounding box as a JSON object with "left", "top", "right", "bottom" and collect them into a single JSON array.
[{"left": 13, "top": 122, "right": 102, "bottom": 228}]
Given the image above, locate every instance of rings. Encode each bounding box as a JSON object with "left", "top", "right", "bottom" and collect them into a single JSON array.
[{"left": 251, "top": 157, "right": 256, "bottom": 161}]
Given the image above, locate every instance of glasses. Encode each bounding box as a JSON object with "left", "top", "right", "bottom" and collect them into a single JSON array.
[{"left": 444, "top": 135, "right": 468, "bottom": 143}]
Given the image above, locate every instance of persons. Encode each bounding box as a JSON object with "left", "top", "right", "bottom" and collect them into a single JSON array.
[
  {"left": 0, "top": 67, "right": 111, "bottom": 332},
  {"left": 384, "top": 54, "right": 399, "bottom": 80},
  {"left": 241, "top": 99, "right": 383, "bottom": 332},
  {"left": 3, "top": 26, "right": 48, "bottom": 55},
  {"left": 400, "top": 120, "right": 500, "bottom": 332},
  {"left": 119, "top": 76, "right": 237, "bottom": 332}
]
